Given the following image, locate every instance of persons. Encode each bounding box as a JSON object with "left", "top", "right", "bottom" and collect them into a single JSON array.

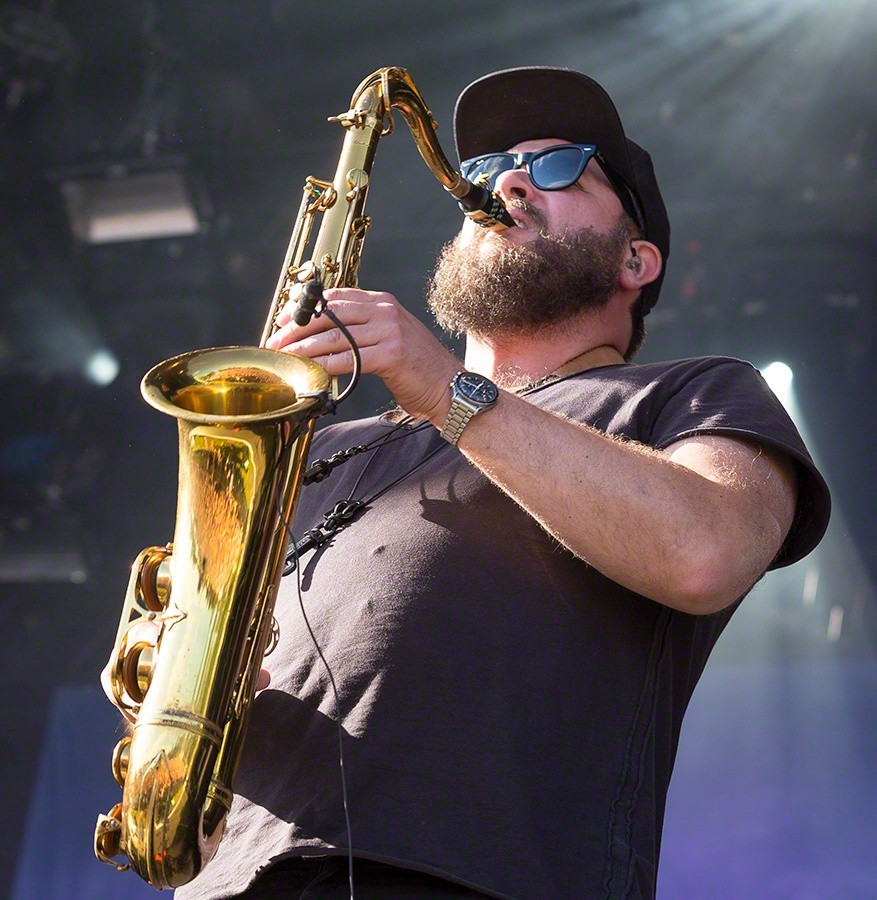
[{"left": 171, "top": 65, "right": 831, "bottom": 900}]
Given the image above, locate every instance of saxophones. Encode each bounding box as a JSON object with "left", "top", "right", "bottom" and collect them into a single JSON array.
[{"left": 92, "top": 66, "right": 518, "bottom": 893}]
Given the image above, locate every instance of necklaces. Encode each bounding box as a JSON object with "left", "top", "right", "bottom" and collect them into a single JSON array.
[{"left": 280, "top": 416, "right": 449, "bottom": 575}]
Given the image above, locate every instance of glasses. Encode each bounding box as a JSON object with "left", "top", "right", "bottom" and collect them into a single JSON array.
[{"left": 460, "top": 143, "right": 642, "bottom": 224}]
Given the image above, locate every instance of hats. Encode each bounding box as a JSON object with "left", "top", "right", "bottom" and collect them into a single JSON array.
[{"left": 453, "top": 67, "right": 670, "bottom": 309}]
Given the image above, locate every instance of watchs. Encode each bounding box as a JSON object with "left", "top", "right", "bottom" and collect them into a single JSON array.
[{"left": 439, "top": 372, "right": 500, "bottom": 448}]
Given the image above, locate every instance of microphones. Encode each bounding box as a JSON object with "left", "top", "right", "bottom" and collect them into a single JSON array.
[{"left": 293, "top": 279, "right": 361, "bottom": 407}]
